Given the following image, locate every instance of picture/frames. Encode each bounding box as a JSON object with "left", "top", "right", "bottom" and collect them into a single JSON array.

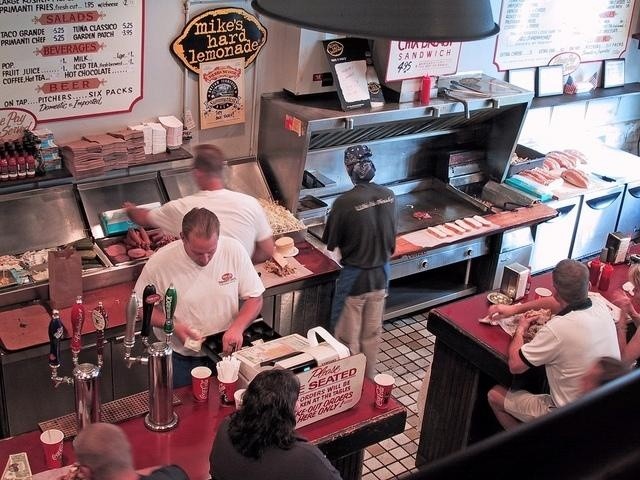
[
  {"left": 508, "top": 68, "right": 535, "bottom": 99},
  {"left": 537, "top": 64, "right": 564, "bottom": 97},
  {"left": 603, "top": 58, "right": 625, "bottom": 89}
]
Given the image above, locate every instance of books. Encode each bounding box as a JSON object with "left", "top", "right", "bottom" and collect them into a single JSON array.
[
  {"left": 322, "top": 38, "right": 386, "bottom": 111},
  {"left": 448, "top": 73, "right": 521, "bottom": 97}
]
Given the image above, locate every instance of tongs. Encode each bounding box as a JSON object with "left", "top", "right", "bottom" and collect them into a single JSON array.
[{"left": 445, "top": 80, "right": 491, "bottom": 98}]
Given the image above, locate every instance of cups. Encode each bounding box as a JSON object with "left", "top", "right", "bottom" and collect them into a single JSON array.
[
  {"left": 40, "top": 430, "right": 65, "bottom": 469},
  {"left": 234, "top": 389, "right": 246, "bottom": 413},
  {"left": 374, "top": 373, "right": 394, "bottom": 410},
  {"left": 191, "top": 366, "right": 212, "bottom": 402},
  {"left": 216, "top": 375, "right": 240, "bottom": 407}
]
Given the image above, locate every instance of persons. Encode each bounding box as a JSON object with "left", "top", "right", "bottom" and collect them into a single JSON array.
[
  {"left": 130, "top": 208, "right": 267, "bottom": 389},
  {"left": 487, "top": 258, "right": 623, "bottom": 433},
  {"left": 323, "top": 144, "right": 397, "bottom": 381},
  {"left": 613, "top": 262, "right": 640, "bottom": 366},
  {"left": 124, "top": 144, "right": 274, "bottom": 268},
  {"left": 56, "top": 421, "right": 189, "bottom": 479},
  {"left": 209, "top": 365, "right": 343, "bottom": 480},
  {"left": 579, "top": 355, "right": 632, "bottom": 405}
]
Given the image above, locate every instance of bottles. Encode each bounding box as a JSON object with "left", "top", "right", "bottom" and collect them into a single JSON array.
[
  {"left": 421, "top": 72, "right": 431, "bottom": 105},
  {"left": 0, "top": 135, "right": 45, "bottom": 180},
  {"left": 598, "top": 264, "right": 614, "bottom": 291},
  {"left": 589, "top": 257, "right": 601, "bottom": 286}
]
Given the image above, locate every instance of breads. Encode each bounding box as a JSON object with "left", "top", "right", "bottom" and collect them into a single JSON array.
[
  {"left": 519, "top": 144, "right": 588, "bottom": 191},
  {"left": 428, "top": 214, "right": 493, "bottom": 239}
]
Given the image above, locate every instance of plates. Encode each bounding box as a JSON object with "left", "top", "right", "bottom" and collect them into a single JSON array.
[
  {"left": 487, "top": 292, "right": 513, "bottom": 305},
  {"left": 535, "top": 287, "right": 552, "bottom": 296},
  {"left": 274, "top": 247, "right": 299, "bottom": 257}
]
